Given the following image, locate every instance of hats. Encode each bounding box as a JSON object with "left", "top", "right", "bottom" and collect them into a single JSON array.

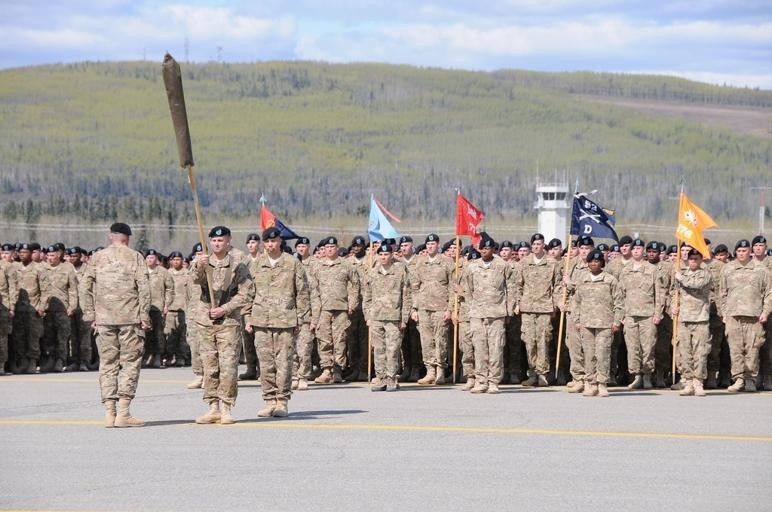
[
  {"left": 262, "top": 227, "right": 460, "bottom": 254},
  {"left": 462, "top": 231, "right": 677, "bottom": 260},
  {"left": 144, "top": 243, "right": 208, "bottom": 268},
  {"left": 111, "top": 222, "right": 131, "bottom": 235},
  {"left": 0, "top": 243, "right": 105, "bottom": 255},
  {"left": 680, "top": 236, "right": 772, "bottom": 260},
  {"left": 208, "top": 226, "right": 231, "bottom": 237},
  {"left": 246, "top": 234, "right": 260, "bottom": 245}
]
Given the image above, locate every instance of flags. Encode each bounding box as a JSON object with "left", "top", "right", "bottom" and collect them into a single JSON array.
[
  {"left": 260, "top": 205, "right": 299, "bottom": 239},
  {"left": 677, "top": 194, "right": 718, "bottom": 260},
  {"left": 456, "top": 193, "right": 485, "bottom": 242},
  {"left": 571, "top": 187, "right": 619, "bottom": 242},
  {"left": 367, "top": 199, "right": 402, "bottom": 240}
]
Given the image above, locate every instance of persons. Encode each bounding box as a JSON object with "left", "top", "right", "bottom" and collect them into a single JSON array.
[
  {"left": 311, "top": 234, "right": 771, "bottom": 394},
  {"left": 240, "top": 227, "right": 310, "bottom": 417},
  {"left": 76, "top": 223, "right": 152, "bottom": 428},
  {"left": 189, "top": 224, "right": 255, "bottom": 424},
  {"left": 1, "top": 236, "right": 311, "bottom": 394}
]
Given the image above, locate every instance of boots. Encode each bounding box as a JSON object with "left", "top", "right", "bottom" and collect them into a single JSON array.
[
  {"left": 141, "top": 354, "right": 191, "bottom": 368},
  {"left": 104, "top": 398, "right": 146, "bottom": 428},
  {"left": 195, "top": 400, "right": 221, "bottom": 423},
  {"left": 291, "top": 364, "right": 467, "bottom": 392},
  {"left": 0, "top": 357, "right": 101, "bottom": 375},
  {"left": 239, "top": 366, "right": 257, "bottom": 379},
  {"left": 566, "top": 368, "right": 672, "bottom": 396},
  {"left": 257, "top": 399, "right": 288, "bottom": 418},
  {"left": 219, "top": 400, "right": 235, "bottom": 424},
  {"left": 463, "top": 366, "right": 566, "bottom": 392},
  {"left": 187, "top": 374, "right": 204, "bottom": 388},
  {"left": 670, "top": 371, "right": 772, "bottom": 395}
]
